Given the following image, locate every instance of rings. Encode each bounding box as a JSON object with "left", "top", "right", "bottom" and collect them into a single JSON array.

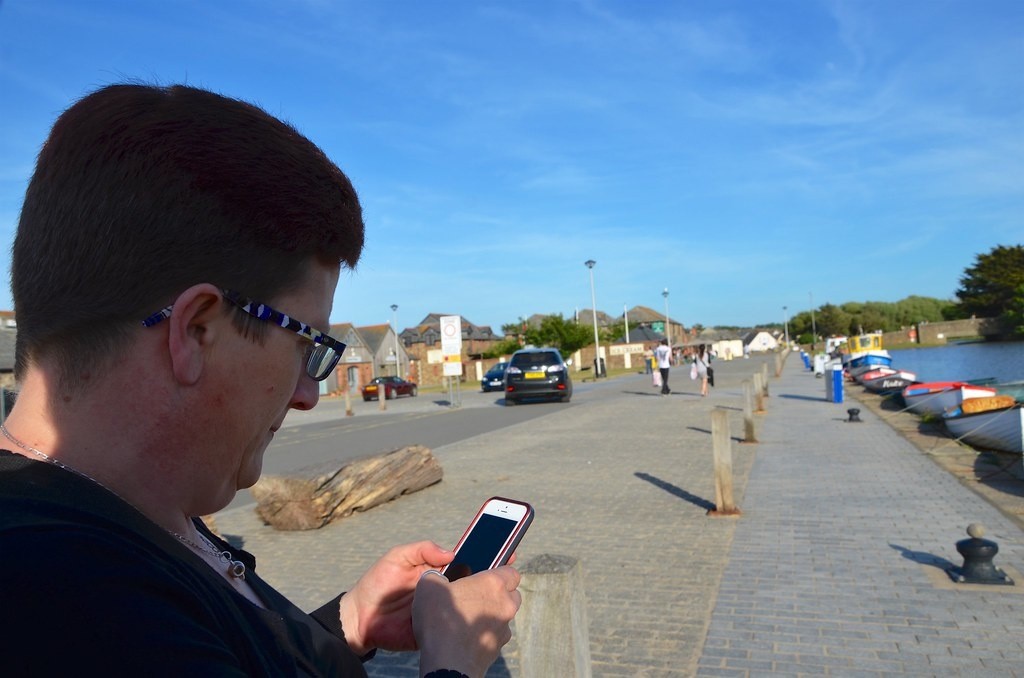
[{"left": 422, "top": 570, "right": 441, "bottom": 577}]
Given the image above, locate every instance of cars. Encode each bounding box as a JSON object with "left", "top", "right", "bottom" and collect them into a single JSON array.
[
  {"left": 481, "top": 361, "right": 510, "bottom": 392},
  {"left": 503, "top": 347, "right": 571, "bottom": 403},
  {"left": 361, "top": 376, "right": 418, "bottom": 399}
]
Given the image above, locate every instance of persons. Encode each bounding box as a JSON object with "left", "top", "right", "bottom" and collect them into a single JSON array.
[
  {"left": 656, "top": 339, "right": 673, "bottom": 395},
  {"left": 694, "top": 344, "right": 711, "bottom": 397},
  {"left": 744, "top": 344, "right": 749, "bottom": 355},
  {"left": 672, "top": 348, "right": 687, "bottom": 366},
  {"left": 0, "top": 84, "right": 522, "bottom": 678},
  {"left": 643, "top": 347, "right": 654, "bottom": 374}
]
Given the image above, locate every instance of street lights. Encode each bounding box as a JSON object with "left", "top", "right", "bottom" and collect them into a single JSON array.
[
  {"left": 661, "top": 287, "right": 672, "bottom": 348},
  {"left": 391, "top": 304, "right": 401, "bottom": 379},
  {"left": 783, "top": 305, "right": 790, "bottom": 347},
  {"left": 584, "top": 259, "right": 606, "bottom": 378}
]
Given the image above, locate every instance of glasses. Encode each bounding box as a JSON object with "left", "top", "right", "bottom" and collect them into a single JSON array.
[{"left": 142, "top": 287, "right": 347, "bottom": 383}]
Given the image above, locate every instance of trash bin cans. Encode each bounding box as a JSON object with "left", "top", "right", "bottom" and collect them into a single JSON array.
[
  {"left": 803, "top": 353, "right": 810, "bottom": 368},
  {"left": 823, "top": 362, "right": 843, "bottom": 402},
  {"left": 800, "top": 349, "right": 804, "bottom": 360},
  {"left": 594, "top": 357, "right": 606, "bottom": 378}
]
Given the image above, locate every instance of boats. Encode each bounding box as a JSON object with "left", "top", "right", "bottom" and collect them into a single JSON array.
[
  {"left": 897, "top": 377, "right": 1024, "bottom": 418},
  {"left": 940, "top": 395, "right": 1024, "bottom": 456},
  {"left": 861, "top": 367, "right": 924, "bottom": 396},
  {"left": 823, "top": 331, "right": 897, "bottom": 384}
]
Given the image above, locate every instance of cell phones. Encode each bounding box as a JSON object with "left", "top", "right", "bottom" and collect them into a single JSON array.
[{"left": 439, "top": 496, "right": 535, "bottom": 583}]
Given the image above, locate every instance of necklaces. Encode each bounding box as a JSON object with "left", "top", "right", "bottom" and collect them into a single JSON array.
[{"left": 0, "top": 423, "right": 246, "bottom": 580}]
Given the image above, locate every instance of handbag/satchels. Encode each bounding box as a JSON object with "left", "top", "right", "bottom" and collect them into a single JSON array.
[
  {"left": 707, "top": 367, "right": 714, "bottom": 376},
  {"left": 690, "top": 367, "right": 697, "bottom": 379},
  {"left": 652, "top": 370, "right": 662, "bottom": 387}
]
